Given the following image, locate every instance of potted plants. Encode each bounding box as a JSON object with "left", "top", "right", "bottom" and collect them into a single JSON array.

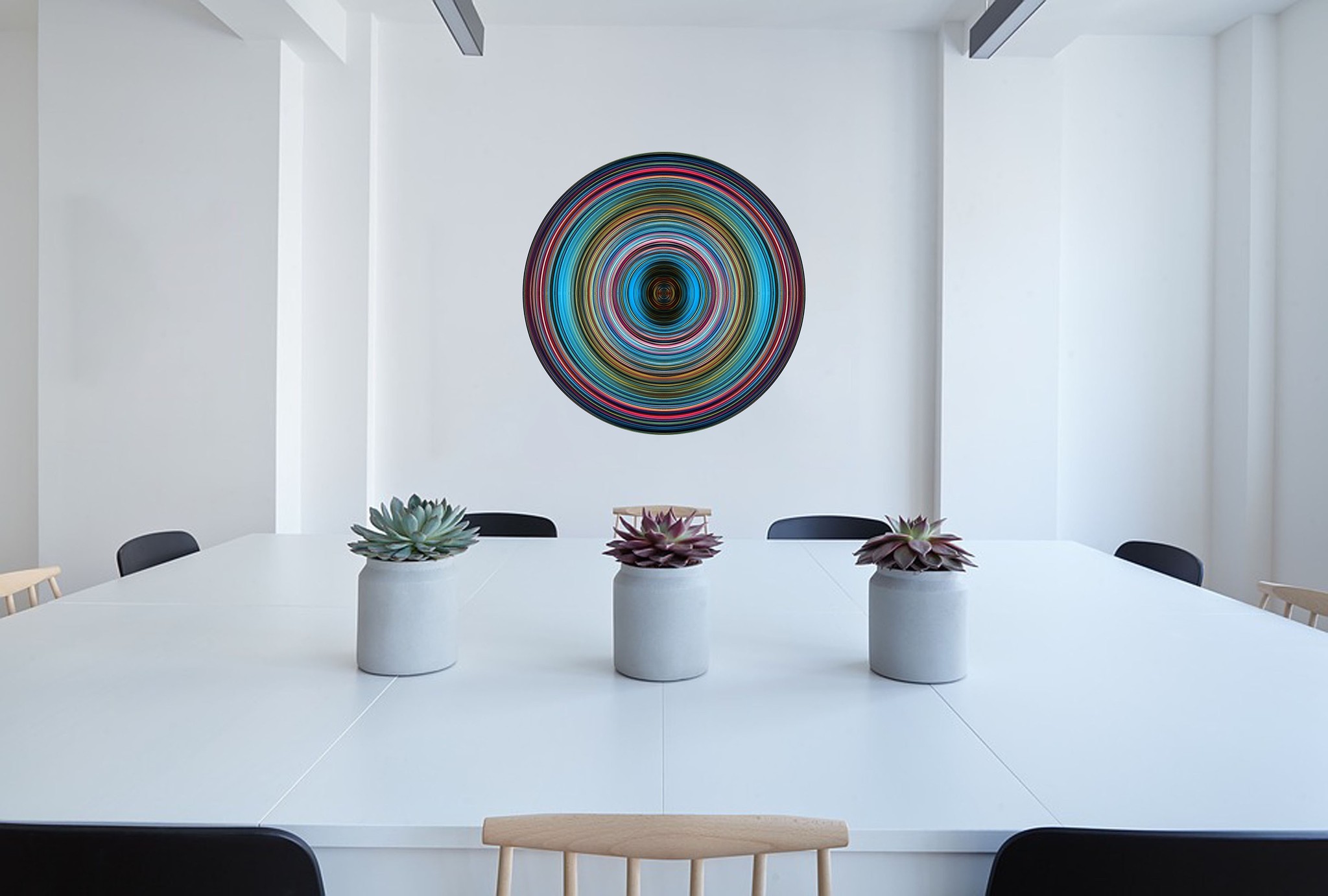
[
  {"left": 346, "top": 493, "right": 480, "bottom": 675},
  {"left": 602, "top": 506, "right": 723, "bottom": 681},
  {"left": 853, "top": 513, "right": 979, "bottom": 683}
]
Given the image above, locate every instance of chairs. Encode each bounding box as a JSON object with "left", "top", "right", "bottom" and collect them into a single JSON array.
[
  {"left": 116, "top": 531, "right": 200, "bottom": 576},
  {"left": 482, "top": 815, "right": 850, "bottom": 896},
  {"left": 0, "top": 821, "right": 326, "bottom": 896},
  {"left": 0, "top": 566, "right": 60, "bottom": 615},
  {"left": 1115, "top": 540, "right": 1204, "bottom": 588},
  {"left": 768, "top": 516, "right": 893, "bottom": 540},
  {"left": 458, "top": 512, "right": 558, "bottom": 538},
  {"left": 1257, "top": 581, "right": 1328, "bottom": 632},
  {"left": 612, "top": 506, "right": 712, "bottom": 538},
  {"left": 983, "top": 827, "right": 1328, "bottom": 896}
]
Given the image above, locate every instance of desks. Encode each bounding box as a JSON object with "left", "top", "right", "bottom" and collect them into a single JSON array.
[{"left": 0, "top": 533, "right": 1328, "bottom": 896}]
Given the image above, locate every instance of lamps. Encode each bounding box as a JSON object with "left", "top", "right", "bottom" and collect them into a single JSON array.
[
  {"left": 969, "top": 0, "right": 1046, "bottom": 59},
  {"left": 433, "top": 0, "right": 485, "bottom": 56}
]
